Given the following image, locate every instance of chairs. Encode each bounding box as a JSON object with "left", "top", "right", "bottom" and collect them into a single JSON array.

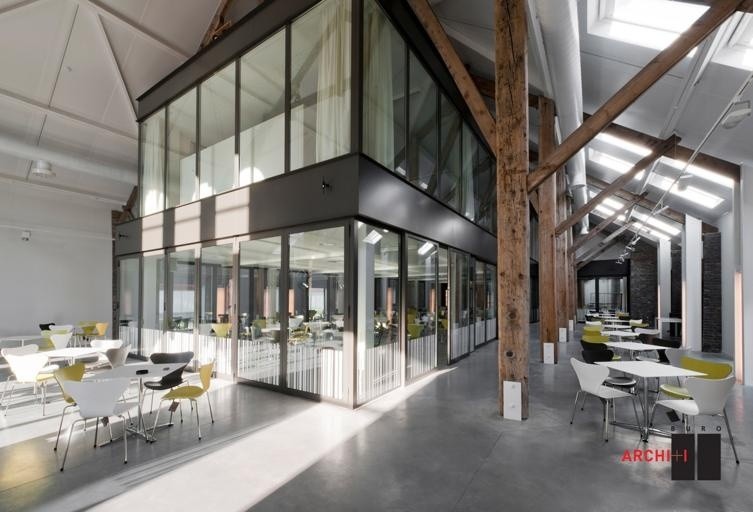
[
  {"left": 0, "top": 320, "right": 217, "bottom": 472},
  {"left": 569, "top": 309, "right": 740, "bottom": 465},
  {"left": 172, "top": 307, "right": 448, "bottom": 350}
]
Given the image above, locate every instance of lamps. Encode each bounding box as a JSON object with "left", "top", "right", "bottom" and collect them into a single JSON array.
[{"left": 721, "top": 95, "right": 753, "bottom": 129}]
